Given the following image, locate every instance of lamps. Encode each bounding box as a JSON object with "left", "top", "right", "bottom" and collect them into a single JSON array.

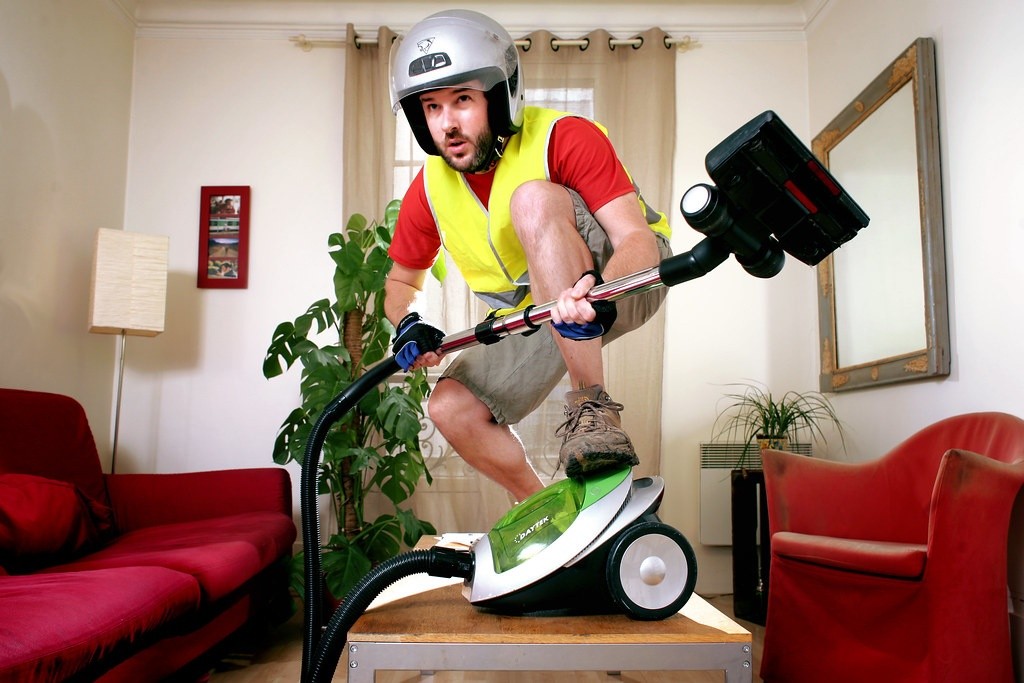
[{"left": 88, "top": 227, "right": 169, "bottom": 475}]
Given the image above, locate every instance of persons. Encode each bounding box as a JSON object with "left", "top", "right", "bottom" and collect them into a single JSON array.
[
  {"left": 383, "top": 10, "right": 673, "bottom": 504},
  {"left": 209, "top": 197, "right": 239, "bottom": 278}
]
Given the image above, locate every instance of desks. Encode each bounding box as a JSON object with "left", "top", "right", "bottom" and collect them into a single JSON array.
[{"left": 347, "top": 534, "right": 754, "bottom": 683}]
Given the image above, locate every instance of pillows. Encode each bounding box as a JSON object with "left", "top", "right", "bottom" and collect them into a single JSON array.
[{"left": 0, "top": 474, "right": 113, "bottom": 575}]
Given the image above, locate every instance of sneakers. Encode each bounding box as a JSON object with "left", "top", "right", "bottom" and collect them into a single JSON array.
[{"left": 550, "top": 381, "right": 640, "bottom": 480}]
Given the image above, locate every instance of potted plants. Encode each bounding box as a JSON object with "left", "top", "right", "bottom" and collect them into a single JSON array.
[
  {"left": 258, "top": 200, "right": 450, "bottom": 620},
  {"left": 698, "top": 378, "right": 847, "bottom": 484}
]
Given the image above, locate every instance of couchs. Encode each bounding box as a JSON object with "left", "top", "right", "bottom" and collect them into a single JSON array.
[
  {"left": 0, "top": 387, "right": 297, "bottom": 683},
  {"left": 760, "top": 412, "right": 1024, "bottom": 683}
]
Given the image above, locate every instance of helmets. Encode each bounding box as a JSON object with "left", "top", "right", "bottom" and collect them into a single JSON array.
[{"left": 388, "top": 9, "right": 525, "bottom": 156}]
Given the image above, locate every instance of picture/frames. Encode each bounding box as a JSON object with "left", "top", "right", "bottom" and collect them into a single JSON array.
[{"left": 196, "top": 185, "right": 250, "bottom": 290}]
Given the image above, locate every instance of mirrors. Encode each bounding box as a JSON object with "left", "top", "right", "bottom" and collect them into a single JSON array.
[{"left": 811, "top": 37, "right": 951, "bottom": 395}]
